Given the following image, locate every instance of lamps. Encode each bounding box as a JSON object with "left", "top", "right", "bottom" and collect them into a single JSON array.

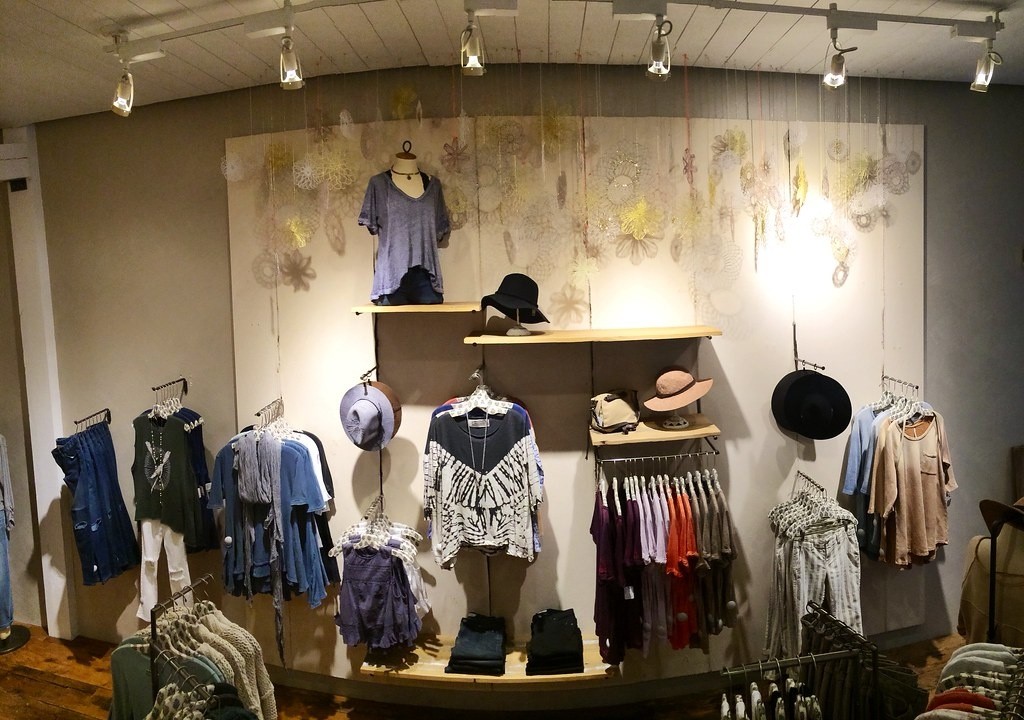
[
  {"left": 279, "top": 27, "right": 306, "bottom": 90},
  {"left": 110, "top": 64, "right": 134, "bottom": 118},
  {"left": 460, "top": 11, "right": 487, "bottom": 76},
  {"left": 646, "top": 16, "right": 673, "bottom": 82},
  {"left": 970, "top": 39, "right": 1003, "bottom": 92},
  {"left": 815, "top": 29, "right": 858, "bottom": 91}
]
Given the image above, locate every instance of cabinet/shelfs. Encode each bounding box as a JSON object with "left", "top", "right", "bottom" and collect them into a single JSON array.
[{"left": 352, "top": 301, "right": 723, "bottom": 447}]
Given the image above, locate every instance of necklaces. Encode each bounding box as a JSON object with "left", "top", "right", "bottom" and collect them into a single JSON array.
[
  {"left": 391, "top": 165, "right": 420, "bottom": 180},
  {"left": 151, "top": 413, "right": 162, "bottom": 505},
  {"left": 466, "top": 408, "right": 488, "bottom": 490}
]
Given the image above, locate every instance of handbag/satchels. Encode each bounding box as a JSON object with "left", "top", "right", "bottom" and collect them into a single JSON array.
[{"left": 587, "top": 388, "right": 640, "bottom": 434}]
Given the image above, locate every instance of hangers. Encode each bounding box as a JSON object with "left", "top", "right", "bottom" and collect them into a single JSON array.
[
  {"left": 328, "top": 495, "right": 423, "bottom": 566},
  {"left": 768, "top": 472, "right": 858, "bottom": 539},
  {"left": 232, "top": 398, "right": 303, "bottom": 449},
  {"left": 598, "top": 450, "right": 720, "bottom": 515},
  {"left": 436, "top": 369, "right": 512, "bottom": 418},
  {"left": 865, "top": 375, "right": 934, "bottom": 424},
  {"left": 131, "top": 380, "right": 206, "bottom": 434},
  {"left": 131, "top": 572, "right": 217, "bottom": 720},
  {"left": 721, "top": 652, "right": 822, "bottom": 720},
  {"left": 935, "top": 646, "right": 1024, "bottom": 720}
]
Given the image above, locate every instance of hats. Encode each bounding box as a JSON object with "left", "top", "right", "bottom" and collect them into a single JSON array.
[
  {"left": 481, "top": 273, "right": 550, "bottom": 324},
  {"left": 644, "top": 370, "right": 713, "bottom": 411},
  {"left": 771, "top": 369, "right": 852, "bottom": 439},
  {"left": 358, "top": 381, "right": 401, "bottom": 439},
  {"left": 340, "top": 384, "right": 394, "bottom": 452}
]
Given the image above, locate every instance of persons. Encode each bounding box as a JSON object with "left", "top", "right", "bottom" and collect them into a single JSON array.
[
  {"left": 0, "top": 434, "right": 15, "bottom": 639},
  {"left": 357, "top": 152, "right": 451, "bottom": 306},
  {"left": 956, "top": 505, "right": 1024, "bottom": 648}
]
